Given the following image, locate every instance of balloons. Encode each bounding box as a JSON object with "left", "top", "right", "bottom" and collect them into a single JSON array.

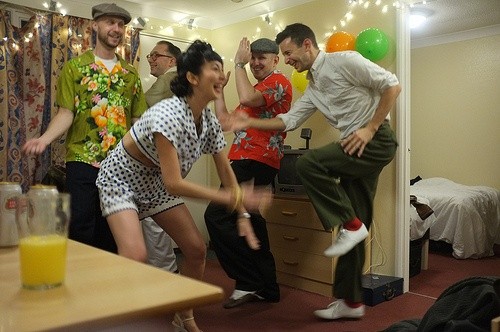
[
  {"left": 289, "top": 69, "right": 310, "bottom": 94},
  {"left": 325, "top": 31, "right": 356, "bottom": 53},
  {"left": 355, "top": 28, "right": 389, "bottom": 62}
]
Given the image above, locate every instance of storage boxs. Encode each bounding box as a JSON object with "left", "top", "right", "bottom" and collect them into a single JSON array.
[{"left": 359, "top": 273, "right": 404, "bottom": 307}]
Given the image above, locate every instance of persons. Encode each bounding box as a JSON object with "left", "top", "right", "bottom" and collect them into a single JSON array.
[
  {"left": 95, "top": 39, "right": 271, "bottom": 332},
  {"left": 140, "top": 41, "right": 180, "bottom": 275},
  {"left": 217, "top": 23, "right": 401, "bottom": 319},
  {"left": 204, "top": 37, "right": 293, "bottom": 308},
  {"left": 21, "top": 3, "right": 149, "bottom": 255}
]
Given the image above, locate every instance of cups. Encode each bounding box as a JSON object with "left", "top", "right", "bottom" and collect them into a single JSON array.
[{"left": 13, "top": 191, "right": 72, "bottom": 289}]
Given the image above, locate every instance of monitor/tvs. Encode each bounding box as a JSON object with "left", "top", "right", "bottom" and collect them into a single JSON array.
[{"left": 274, "top": 150, "right": 309, "bottom": 195}]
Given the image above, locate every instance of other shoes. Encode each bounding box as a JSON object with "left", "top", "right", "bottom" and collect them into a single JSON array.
[
  {"left": 171, "top": 313, "right": 194, "bottom": 332},
  {"left": 223, "top": 282, "right": 280, "bottom": 309}
]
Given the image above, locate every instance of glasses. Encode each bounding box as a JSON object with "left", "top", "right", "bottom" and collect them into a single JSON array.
[{"left": 146, "top": 51, "right": 172, "bottom": 62}]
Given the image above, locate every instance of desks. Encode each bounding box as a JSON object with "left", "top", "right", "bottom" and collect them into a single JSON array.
[{"left": 0, "top": 239, "right": 225, "bottom": 332}]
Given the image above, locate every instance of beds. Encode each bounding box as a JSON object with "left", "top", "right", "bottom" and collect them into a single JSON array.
[{"left": 410, "top": 176, "right": 500, "bottom": 280}]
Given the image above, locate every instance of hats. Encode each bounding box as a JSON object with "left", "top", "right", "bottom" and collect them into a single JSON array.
[
  {"left": 92, "top": 3, "right": 131, "bottom": 26},
  {"left": 250, "top": 38, "right": 279, "bottom": 55}
]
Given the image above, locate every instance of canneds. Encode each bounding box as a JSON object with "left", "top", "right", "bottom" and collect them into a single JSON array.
[
  {"left": 0, "top": 182, "right": 24, "bottom": 247},
  {"left": 25, "top": 183, "right": 58, "bottom": 234}
]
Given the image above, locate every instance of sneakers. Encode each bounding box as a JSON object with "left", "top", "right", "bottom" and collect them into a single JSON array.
[
  {"left": 314, "top": 299, "right": 365, "bottom": 319},
  {"left": 323, "top": 223, "right": 368, "bottom": 257}
]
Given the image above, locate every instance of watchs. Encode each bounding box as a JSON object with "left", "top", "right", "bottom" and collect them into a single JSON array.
[{"left": 236, "top": 213, "right": 251, "bottom": 218}]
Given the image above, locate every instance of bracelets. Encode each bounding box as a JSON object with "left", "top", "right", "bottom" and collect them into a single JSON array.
[
  {"left": 235, "top": 63, "right": 245, "bottom": 68},
  {"left": 228, "top": 183, "right": 242, "bottom": 214}
]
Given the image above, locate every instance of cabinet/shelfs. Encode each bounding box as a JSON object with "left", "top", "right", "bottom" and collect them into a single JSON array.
[{"left": 266, "top": 197, "right": 371, "bottom": 298}]
